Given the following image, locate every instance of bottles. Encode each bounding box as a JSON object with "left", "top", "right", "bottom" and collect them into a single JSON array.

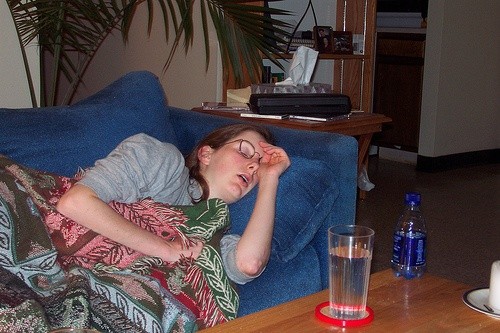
[{"left": 391, "top": 192, "right": 427, "bottom": 280}]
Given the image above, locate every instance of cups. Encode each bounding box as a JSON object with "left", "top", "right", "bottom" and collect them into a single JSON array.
[
  {"left": 488, "top": 260, "right": 500, "bottom": 310},
  {"left": 328, "top": 224, "right": 375, "bottom": 320}
]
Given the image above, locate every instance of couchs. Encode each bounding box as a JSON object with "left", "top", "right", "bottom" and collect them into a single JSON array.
[{"left": 0, "top": 71, "right": 358, "bottom": 318}]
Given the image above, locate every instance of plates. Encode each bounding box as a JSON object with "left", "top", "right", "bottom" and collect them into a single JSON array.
[{"left": 460, "top": 287, "right": 500, "bottom": 320}]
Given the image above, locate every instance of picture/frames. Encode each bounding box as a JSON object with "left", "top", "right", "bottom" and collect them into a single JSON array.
[
  {"left": 313, "top": 26, "right": 334, "bottom": 54},
  {"left": 333, "top": 31, "right": 353, "bottom": 55}
]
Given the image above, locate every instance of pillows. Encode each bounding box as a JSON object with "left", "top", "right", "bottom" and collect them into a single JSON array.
[{"left": 226, "top": 157, "right": 339, "bottom": 263}]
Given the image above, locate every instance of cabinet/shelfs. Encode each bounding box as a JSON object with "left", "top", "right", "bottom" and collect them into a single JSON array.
[
  {"left": 222, "top": 0, "right": 377, "bottom": 113},
  {"left": 371, "top": 40, "right": 426, "bottom": 166}
]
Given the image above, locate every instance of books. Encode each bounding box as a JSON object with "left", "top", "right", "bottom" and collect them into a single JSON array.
[
  {"left": 289, "top": 115, "right": 349, "bottom": 122},
  {"left": 240, "top": 111, "right": 289, "bottom": 120},
  {"left": 266, "top": 39, "right": 316, "bottom": 54},
  {"left": 201, "top": 86, "right": 251, "bottom": 111}
]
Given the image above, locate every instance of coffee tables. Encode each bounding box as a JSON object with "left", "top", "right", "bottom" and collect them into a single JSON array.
[{"left": 194, "top": 269, "right": 500, "bottom": 333}]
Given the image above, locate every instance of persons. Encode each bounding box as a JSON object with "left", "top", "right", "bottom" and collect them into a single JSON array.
[
  {"left": 0, "top": 124, "right": 291, "bottom": 333},
  {"left": 317, "top": 29, "right": 330, "bottom": 51}
]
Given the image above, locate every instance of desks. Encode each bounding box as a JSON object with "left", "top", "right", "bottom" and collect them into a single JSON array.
[{"left": 191, "top": 107, "right": 389, "bottom": 201}]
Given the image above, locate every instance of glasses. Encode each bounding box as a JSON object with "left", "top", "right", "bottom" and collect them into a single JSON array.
[{"left": 212, "top": 139, "right": 263, "bottom": 165}]
